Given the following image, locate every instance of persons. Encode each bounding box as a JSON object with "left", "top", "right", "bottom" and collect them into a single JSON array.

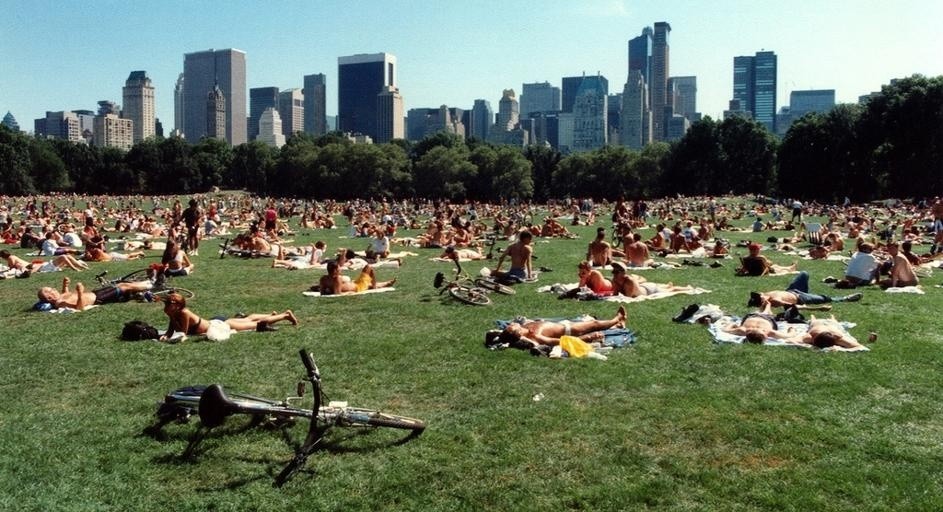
[
  {"left": 798, "top": 314, "right": 859, "bottom": 348},
  {"left": 198, "top": 183, "right": 308, "bottom": 253},
  {"left": 448, "top": 189, "right": 520, "bottom": 247},
  {"left": 612, "top": 264, "right": 693, "bottom": 297},
  {"left": 587, "top": 190, "right": 733, "bottom": 266},
  {"left": 321, "top": 261, "right": 395, "bottom": 293},
  {"left": 504, "top": 305, "right": 628, "bottom": 344},
  {"left": 336, "top": 246, "right": 381, "bottom": 269},
  {"left": 0, "top": 190, "right": 168, "bottom": 273},
  {"left": 38, "top": 269, "right": 156, "bottom": 310},
  {"left": 394, "top": 181, "right": 449, "bottom": 258},
  {"left": 162, "top": 190, "right": 201, "bottom": 277},
  {"left": 579, "top": 261, "right": 646, "bottom": 292},
  {"left": 491, "top": 233, "right": 531, "bottom": 284},
  {"left": 441, "top": 247, "right": 483, "bottom": 263},
  {"left": 798, "top": 196, "right": 943, "bottom": 287},
  {"left": 366, "top": 232, "right": 389, "bottom": 258},
  {"left": 705, "top": 299, "right": 795, "bottom": 344},
  {"left": 732, "top": 192, "right": 803, "bottom": 251},
  {"left": 158, "top": 294, "right": 296, "bottom": 342},
  {"left": 299, "top": 188, "right": 395, "bottom": 237},
  {"left": 735, "top": 243, "right": 797, "bottom": 275},
  {"left": 519, "top": 191, "right": 595, "bottom": 239},
  {"left": 749, "top": 271, "right": 863, "bottom": 310},
  {"left": 271, "top": 240, "right": 326, "bottom": 270}
]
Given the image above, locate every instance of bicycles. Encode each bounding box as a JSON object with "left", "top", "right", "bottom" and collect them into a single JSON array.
[
  {"left": 158, "top": 347, "right": 428, "bottom": 482},
  {"left": 611, "top": 222, "right": 623, "bottom": 247},
  {"left": 515, "top": 212, "right": 537, "bottom": 226},
  {"left": 91, "top": 268, "right": 196, "bottom": 304},
  {"left": 432, "top": 256, "right": 518, "bottom": 309},
  {"left": 219, "top": 238, "right": 268, "bottom": 260}
]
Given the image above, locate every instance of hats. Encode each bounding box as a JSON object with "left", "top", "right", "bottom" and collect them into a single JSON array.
[
  {"left": 611, "top": 261, "right": 628, "bottom": 272},
  {"left": 747, "top": 242, "right": 763, "bottom": 251}
]
